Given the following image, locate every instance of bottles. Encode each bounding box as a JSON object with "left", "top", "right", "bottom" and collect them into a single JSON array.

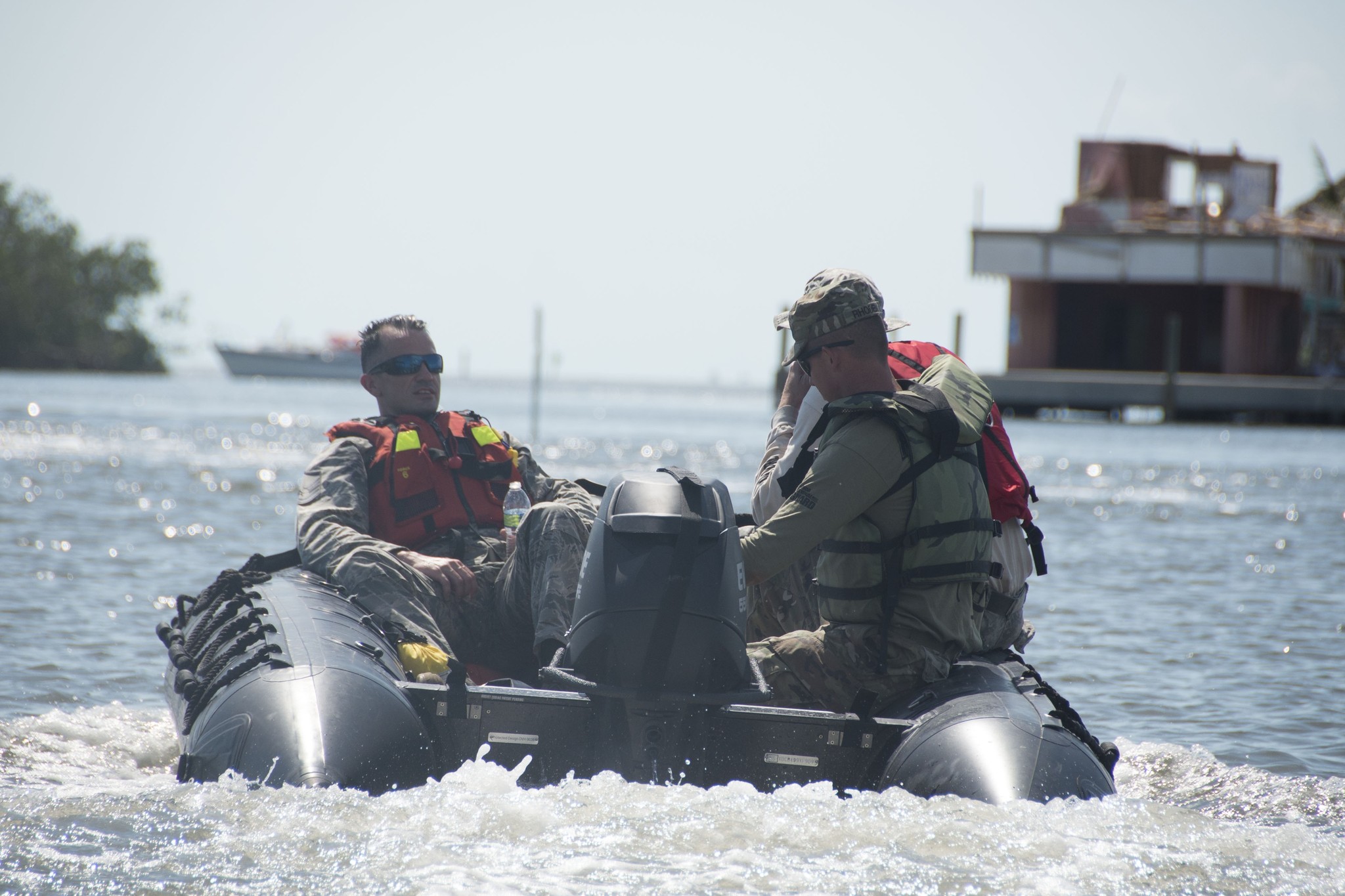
[{"left": 503, "top": 481, "right": 532, "bottom": 561}]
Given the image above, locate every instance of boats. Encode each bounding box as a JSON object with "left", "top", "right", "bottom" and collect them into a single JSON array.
[
  {"left": 208, "top": 339, "right": 377, "bottom": 383},
  {"left": 971, "top": 130, "right": 1344, "bottom": 431},
  {"left": 156, "top": 460, "right": 1121, "bottom": 813}
]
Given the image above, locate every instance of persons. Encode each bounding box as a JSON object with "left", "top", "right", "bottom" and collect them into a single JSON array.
[
  {"left": 733, "top": 269, "right": 1035, "bottom": 658},
  {"left": 738, "top": 278, "right": 1004, "bottom": 714},
  {"left": 297, "top": 315, "right": 598, "bottom": 686}
]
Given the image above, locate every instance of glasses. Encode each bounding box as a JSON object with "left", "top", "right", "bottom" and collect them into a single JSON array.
[
  {"left": 797, "top": 340, "right": 855, "bottom": 376},
  {"left": 362, "top": 354, "right": 443, "bottom": 375}
]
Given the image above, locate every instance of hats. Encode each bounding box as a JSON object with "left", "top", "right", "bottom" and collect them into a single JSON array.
[
  {"left": 781, "top": 268, "right": 884, "bottom": 366},
  {"left": 773, "top": 310, "right": 911, "bottom": 332}
]
